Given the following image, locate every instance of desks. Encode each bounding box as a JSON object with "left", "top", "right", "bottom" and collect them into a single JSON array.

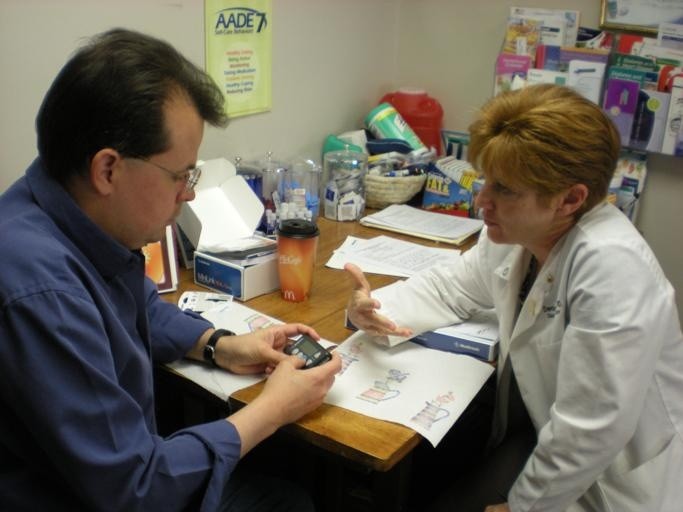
[{"left": 158, "top": 197, "right": 499, "bottom": 512}]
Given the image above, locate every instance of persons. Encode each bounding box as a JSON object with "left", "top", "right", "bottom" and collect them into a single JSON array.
[
  {"left": 344, "top": 84, "right": 682, "bottom": 511},
  {"left": 0, "top": 27, "right": 344, "bottom": 510}
]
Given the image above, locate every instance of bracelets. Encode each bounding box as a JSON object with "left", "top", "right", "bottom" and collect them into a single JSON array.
[{"left": 204, "top": 325, "right": 236, "bottom": 366}]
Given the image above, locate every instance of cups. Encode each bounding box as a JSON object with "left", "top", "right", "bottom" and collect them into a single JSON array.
[
  {"left": 363, "top": 102, "right": 429, "bottom": 159},
  {"left": 274, "top": 218, "right": 321, "bottom": 303}
]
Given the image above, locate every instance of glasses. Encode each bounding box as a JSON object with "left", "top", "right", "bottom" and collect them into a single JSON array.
[{"left": 134, "top": 155, "right": 201, "bottom": 190}]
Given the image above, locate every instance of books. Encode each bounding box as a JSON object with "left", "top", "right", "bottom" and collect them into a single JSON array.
[
  {"left": 359, "top": 202, "right": 484, "bottom": 247},
  {"left": 343, "top": 279, "right": 500, "bottom": 363}
]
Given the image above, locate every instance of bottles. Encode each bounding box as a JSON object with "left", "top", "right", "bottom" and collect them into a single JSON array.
[{"left": 234, "top": 143, "right": 369, "bottom": 223}]
